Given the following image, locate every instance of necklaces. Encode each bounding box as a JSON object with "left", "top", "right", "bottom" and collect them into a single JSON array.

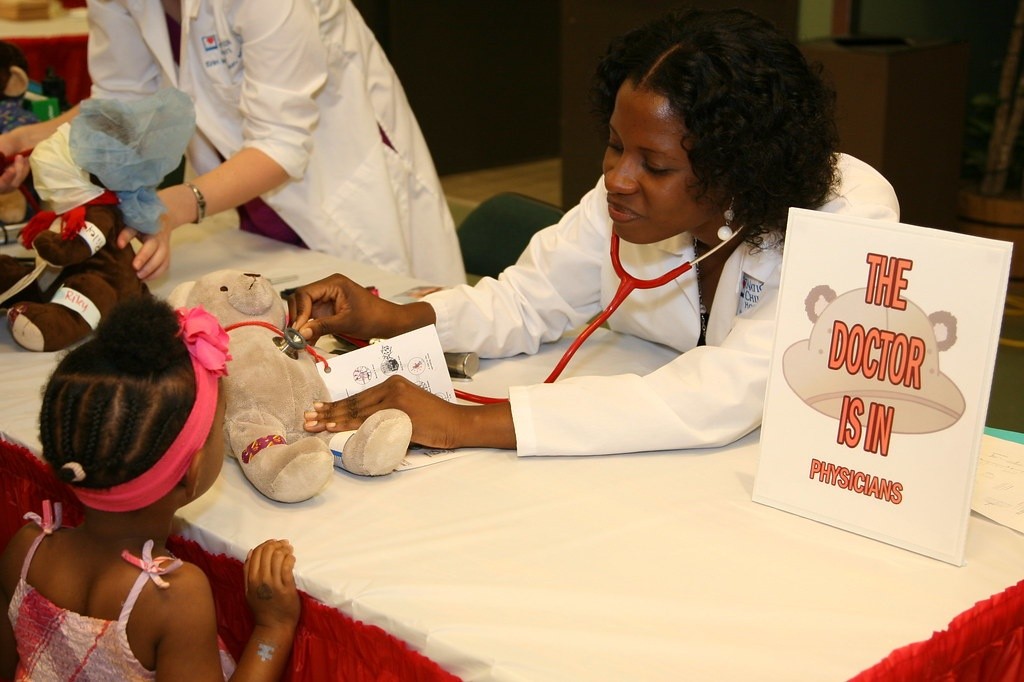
[{"left": 694, "top": 231, "right": 709, "bottom": 339}]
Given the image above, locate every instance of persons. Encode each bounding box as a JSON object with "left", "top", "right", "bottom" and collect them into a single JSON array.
[
  {"left": 1, "top": 296, "right": 306, "bottom": 682},
  {"left": 0, "top": 0, "right": 472, "bottom": 288},
  {"left": 288, "top": 6, "right": 902, "bottom": 458}
]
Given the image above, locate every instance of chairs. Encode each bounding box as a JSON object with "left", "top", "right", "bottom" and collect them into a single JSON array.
[{"left": 454, "top": 191, "right": 571, "bottom": 281}]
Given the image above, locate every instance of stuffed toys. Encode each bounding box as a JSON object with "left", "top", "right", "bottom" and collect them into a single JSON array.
[
  {"left": 167, "top": 270, "right": 412, "bottom": 503},
  {"left": 0, "top": 107, "right": 154, "bottom": 353},
  {"left": 1, "top": 42, "right": 39, "bottom": 131}
]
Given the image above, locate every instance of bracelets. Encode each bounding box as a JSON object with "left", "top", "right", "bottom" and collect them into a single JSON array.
[{"left": 181, "top": 180, "right": 207, "bottom": 226}]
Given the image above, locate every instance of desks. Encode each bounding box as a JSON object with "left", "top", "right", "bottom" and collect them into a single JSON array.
[{"left": 0, "top": 217, "right": 1024, "bottom": 682}]
